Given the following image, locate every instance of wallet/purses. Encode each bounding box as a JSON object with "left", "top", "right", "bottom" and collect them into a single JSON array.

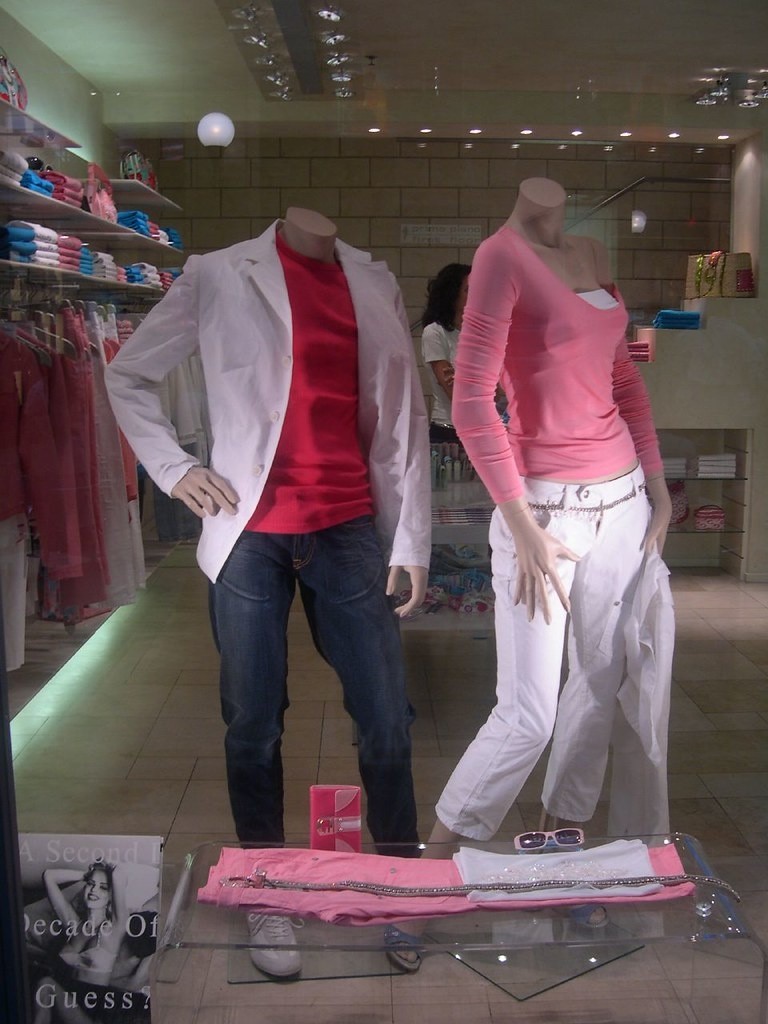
[{"left": 309, "top": 784, "right": 361, "bottom": 852}]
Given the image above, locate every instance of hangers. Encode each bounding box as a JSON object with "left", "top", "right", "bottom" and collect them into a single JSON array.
[{"left": 0, "top": 271, "right": 159, "bottom": 369}]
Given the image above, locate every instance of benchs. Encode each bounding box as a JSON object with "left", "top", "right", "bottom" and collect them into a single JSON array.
[{"left": 151, "top": 835, "right": 767, "bottom": 1023}]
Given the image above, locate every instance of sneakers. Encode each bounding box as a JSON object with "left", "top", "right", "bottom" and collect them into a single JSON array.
[{"left": 246, "top": 913, "right": 306, "bottom": 977}]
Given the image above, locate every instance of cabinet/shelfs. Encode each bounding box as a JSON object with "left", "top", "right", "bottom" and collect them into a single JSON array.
[
  {"left": 660, "top": 474, "right": 747, "bottom": 534},
  {"left": 0, "top": 97, "right": 186, "bottom": 296},
  {"left": 393, "top": 509, "right": 493, "bottom": 631}
]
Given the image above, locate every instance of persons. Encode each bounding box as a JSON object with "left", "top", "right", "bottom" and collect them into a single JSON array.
[
  {"left": 43, "top": 857, "right": 130, "bottom": 974},
  {"left": 384, "top": 176, "right": 672, "bottom": 974},
  {"left": 104, "top": 209, "right": 431, "bottom": 978},
  {"left": 422, "top": 263, "right": 503, "bottom": 485}
]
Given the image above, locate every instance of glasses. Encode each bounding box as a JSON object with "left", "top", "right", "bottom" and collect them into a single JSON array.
[{"left": 515, "top": 828, "right": 584, "bottom": 851}]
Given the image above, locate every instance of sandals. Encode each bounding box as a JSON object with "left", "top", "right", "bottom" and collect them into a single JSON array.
[
  {"left": 560, "top": 902, "right": 611, "bottom": 929},
  {"left": 385, "top": 924, "right": 425, "bottom": 971}
]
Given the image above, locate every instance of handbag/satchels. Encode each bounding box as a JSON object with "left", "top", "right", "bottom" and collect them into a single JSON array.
[
  {"left": 88, "top": 161, "right": 117, "bottom": 224},
  {"left": 686, "top": 252, "right": 755, "bottom": 297},
  {"left": 119, "top": 150, "right": 158, "bottom": 190},
  {"left": 646, "top": 481, "right": 689, "bottom": 524},
  {"left": 0, "top": 49, "right": 28, "bottom": 111},
  {"left": 694, "top": 504, "right": 725, "bottom": 531}
]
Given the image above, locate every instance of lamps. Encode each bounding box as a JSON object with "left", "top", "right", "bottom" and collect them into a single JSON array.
[
  {"left": 214, "top": 0, "right": 363, "bottom": 104},
  {"left": 695, "top": 79, "right": 768, "bottom": 109},
  {"left": 197, "top": 111, "right": 235, "bottom": 161}
]
